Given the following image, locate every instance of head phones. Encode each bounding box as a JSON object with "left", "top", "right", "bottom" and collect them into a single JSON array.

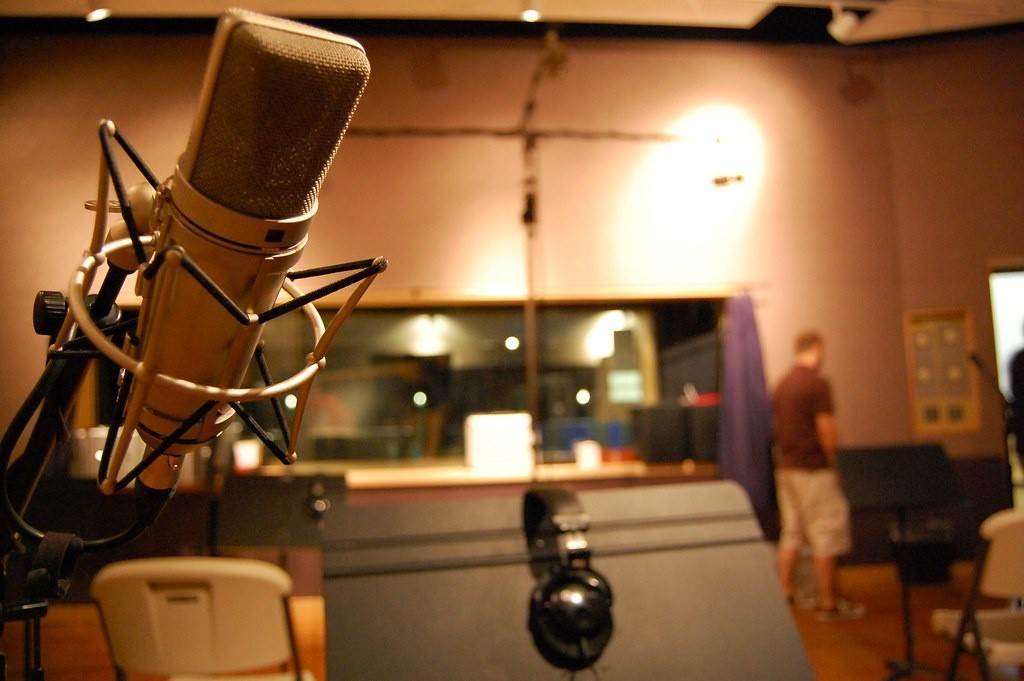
[{"left": 518, "top": 486, "right": 614, "bottom": 669}]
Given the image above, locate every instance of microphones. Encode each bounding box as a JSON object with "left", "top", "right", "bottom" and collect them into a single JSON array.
[{"left": 138, "top": 11, "right": 366, "bottom": 523}]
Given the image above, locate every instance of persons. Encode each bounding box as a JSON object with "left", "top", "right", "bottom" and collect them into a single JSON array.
[
  {"left": 770, "top": 333, "right": 867, "bottom": 620},
  {"left": 373, "top": 355, "right": 450, "bottom": 459}
]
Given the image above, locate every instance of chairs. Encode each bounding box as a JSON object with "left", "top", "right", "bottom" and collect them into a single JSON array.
[{"left": 929, "top": 507, "right": 1024, "bottom": 681}]
